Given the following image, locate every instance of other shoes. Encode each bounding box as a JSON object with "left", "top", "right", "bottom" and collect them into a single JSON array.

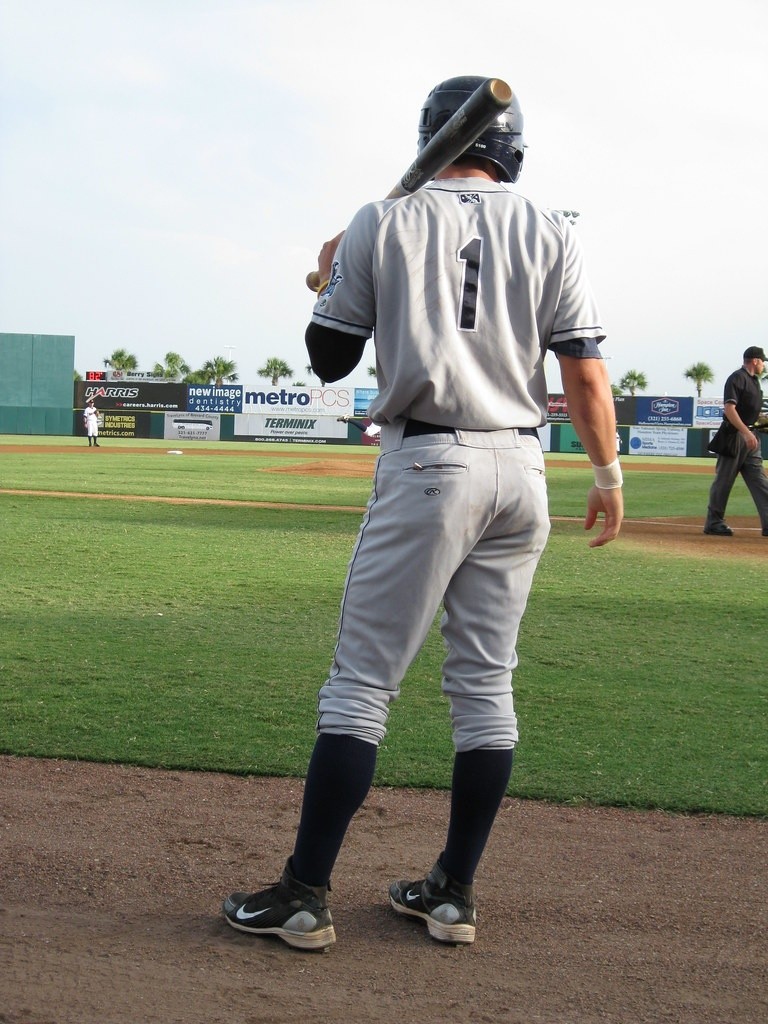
[
  {"left": 89, "top": 442, "right": 100, "bottom": 447},
  {"left": 703, "top": 524, "right": 734, "bottom": 536}
]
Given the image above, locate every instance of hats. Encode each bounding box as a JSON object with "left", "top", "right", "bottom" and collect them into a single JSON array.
[
  {"left": 743, "top": 346, "right": 768, "bottom": 361},
  {"left": 89, "top": 400, "right": 95, "bottom": 403}
]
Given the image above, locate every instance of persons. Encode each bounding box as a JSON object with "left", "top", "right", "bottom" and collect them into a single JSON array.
[
  {"left": 83, "top": 399, "right": 102, "bottom": 447},
  {"left": 222, "top": 76, "right": 628, "bottom": 950},
  {"left": 702, "top": 346, "right": 768, "bottom": 538}
]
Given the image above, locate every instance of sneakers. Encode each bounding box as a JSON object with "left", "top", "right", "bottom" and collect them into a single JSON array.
[
  {"left": 386, "top": 850, "right": 476, "bottom": 945},
  {"left": 221, "top": 854, "right": 338, "bottom": 949}
]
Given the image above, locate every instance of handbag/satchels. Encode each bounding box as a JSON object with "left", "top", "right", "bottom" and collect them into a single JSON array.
[{"left": 705, "top": 421, "right": 740, "bottom": 457}]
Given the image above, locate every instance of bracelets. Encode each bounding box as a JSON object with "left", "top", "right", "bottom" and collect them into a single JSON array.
[{"left": 317, "top": 279, "right": 329, "bottom": 299}]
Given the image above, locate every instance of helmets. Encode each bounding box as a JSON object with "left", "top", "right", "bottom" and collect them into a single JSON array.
[{"left": 418, "top": 76, "right": 525, "bottom": 184}]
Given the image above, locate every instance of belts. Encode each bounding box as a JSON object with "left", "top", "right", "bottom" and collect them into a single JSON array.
[{"left": 402, "top": 419, "right": 539, "bottom": 439}]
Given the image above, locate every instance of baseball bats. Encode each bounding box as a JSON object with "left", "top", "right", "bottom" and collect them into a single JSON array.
[{"left": 305, "top": 77, "right": 514, "bottom": 294}]
